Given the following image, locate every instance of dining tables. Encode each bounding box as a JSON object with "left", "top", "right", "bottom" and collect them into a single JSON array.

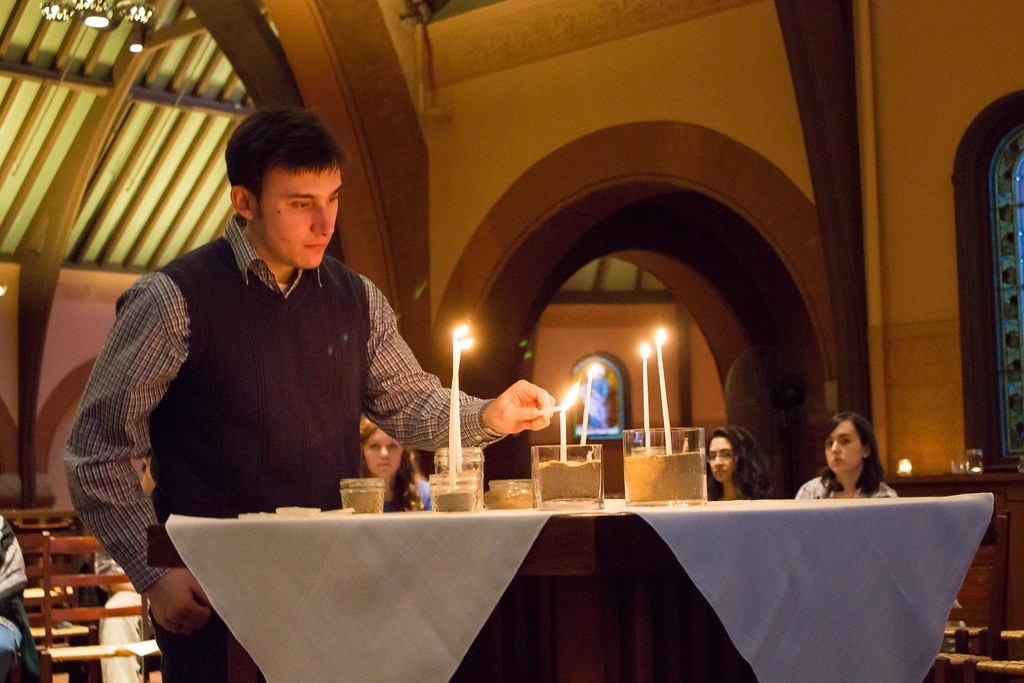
[{"left": 144, "top": 489, "right": 998, "bottom": 683}]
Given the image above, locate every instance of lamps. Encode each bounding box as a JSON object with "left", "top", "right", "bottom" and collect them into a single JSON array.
[{"left": 37, "top": 0, "right": 155, "bottom": 28}]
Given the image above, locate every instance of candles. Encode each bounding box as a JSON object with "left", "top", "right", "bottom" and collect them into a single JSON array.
[
  {"left": 444, "top": 321, "right": 477, "bottom": 485},
  {"left": 533, "top": 360, "right": 604, "bottom": 463},
  {"left": 638, "top": 340, "right": 653, "bottom": 455},
  {"left": 653, "top": 328, "right": 675, "bottom": 455}
]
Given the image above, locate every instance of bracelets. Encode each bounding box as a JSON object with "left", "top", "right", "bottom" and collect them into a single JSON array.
[{"left": 478, "top": 400, "right": 503, "bottom": 437}]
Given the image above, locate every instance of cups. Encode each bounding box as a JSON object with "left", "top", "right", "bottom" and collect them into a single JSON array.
[
  {"left": 338, "top": 476, "right": 386, "bottom": 513},
  {"left": 950, "top": 458, "right": 969, "bottom": 473},
  {"left": 489, "top": 477, "right": 534, "bottom": 509},
  {"left": 965, "top": 449, "right": 983, "bottom": 472}
]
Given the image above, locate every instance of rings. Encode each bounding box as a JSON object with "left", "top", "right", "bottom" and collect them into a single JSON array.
[{"left": 172, "top": 624, "right": 184, "bottom": 634}]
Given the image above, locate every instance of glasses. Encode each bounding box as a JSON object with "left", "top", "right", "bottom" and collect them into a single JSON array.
[{"left": 706, "top": 452, "right": 734, "bottom": 463}]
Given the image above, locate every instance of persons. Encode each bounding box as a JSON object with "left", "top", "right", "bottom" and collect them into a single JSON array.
[
  {"left": 63, "top": 108, "right": 555, "bottom": 682},
  {"left": 0, "top": 514, "right": 41, "bottom": 683},
  {"left": 705, "top": 410, "right": 899, "bottom": 501}
]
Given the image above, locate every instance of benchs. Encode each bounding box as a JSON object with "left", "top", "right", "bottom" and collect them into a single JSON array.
[{"left": 0, "top": 506, "right": 162, "bottom": 683}]
